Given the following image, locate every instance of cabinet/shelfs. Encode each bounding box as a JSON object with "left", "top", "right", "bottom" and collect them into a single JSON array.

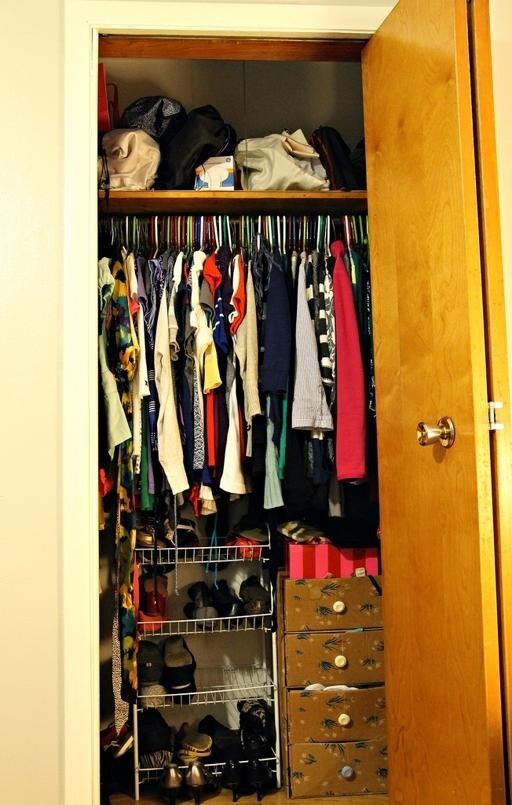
[
  {"left": 277, "top": 571, "right": 388, "bottom": 800},
  {"left": 124, "top": 523, "right": 281, "bottom": 800}
]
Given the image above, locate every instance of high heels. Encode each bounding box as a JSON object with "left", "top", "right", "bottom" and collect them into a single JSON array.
[
  {"left": 239, "top": 574, "right": 270, "bottom": 629},
  {"left": 188, "top": 762, "right": 220, "bottom": 805},
  {"left": 164, "top": 765, "right": 183, "bottom": 804},
  {"left": 247, "top": 760, "right": 269, "bottom": 801},
  {"left": 224, "top": 760, "right": 242, "bottom": 801}
]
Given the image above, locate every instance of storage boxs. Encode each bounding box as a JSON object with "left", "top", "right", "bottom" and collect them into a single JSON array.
[{"left": 284, "top": 542, "right": 379, "bottom": 580}]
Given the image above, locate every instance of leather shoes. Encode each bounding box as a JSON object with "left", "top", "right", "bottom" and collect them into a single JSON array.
[
  {"left": 212, "top": 579, "right": 244, "bottom": 629},
  {"left": 143, "top": 575, "right": 168, "bottom": 616},
  {"left": 183, "top": 582, "right": 218, "bottom": 630}
]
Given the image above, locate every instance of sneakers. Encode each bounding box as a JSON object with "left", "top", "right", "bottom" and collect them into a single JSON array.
[
  {"left": 138, "top": 640, "right": 166, "bottom": 686},
  {"left": 176, "top": 722, "right": 212, "bottom": 765},
  {"left": 163, "top": 636, "right": 196, "bottom": 704}
]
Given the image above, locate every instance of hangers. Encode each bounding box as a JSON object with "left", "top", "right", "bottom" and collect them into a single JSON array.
[{"left": 106, "top": 212, "right": 369, "bottom": 264}]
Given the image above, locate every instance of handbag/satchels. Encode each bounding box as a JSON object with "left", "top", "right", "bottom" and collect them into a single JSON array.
[
  {"left": 235, "top": 129, "right": 331, "bottom": 191},
  {"left": 349, "top": 136, "right": 367, "bottom": 191},
  {"left": 308, "top": 125, "right": 353, "bottom": 189},
  {"left": 98, "top": 129, "right": 161, "bottom": 192},
  {"left": 155, "top": 105, "right": 236, "bottom": 190},
  {"left": 226, "top": 536, "right": 261, "bottom": 559},
  {"left": 122, "top": 96, "right": 183, "bottom": 138}
]
